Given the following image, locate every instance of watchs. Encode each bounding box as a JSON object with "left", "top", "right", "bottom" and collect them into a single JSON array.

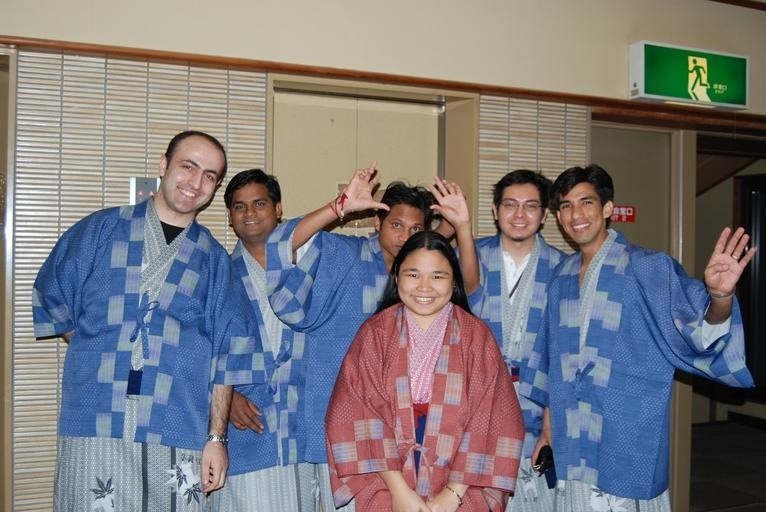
[{"left": 206, "top": 434, "right": 230, "bottom": 444}]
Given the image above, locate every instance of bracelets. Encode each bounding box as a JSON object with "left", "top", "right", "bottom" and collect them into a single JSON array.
[
  {"left": 705, "top": 282, "right": 737, "bottom": 300},
  {"left": 329, "top": 193, "right": 350, "bottom": 224},
  {"left": 442, "top": 480, "right": 466, "bottom": 506}
]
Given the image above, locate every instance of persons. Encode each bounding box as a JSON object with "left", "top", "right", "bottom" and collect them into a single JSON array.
[
  {"left": 324, "top": 229, "right": 526, "bottom": 511},
  {"left": 31, "top": 129, "right": 267, "bottom": 512},
  {"left": 454, "top": 168, "right": 570, "bottom": 512},
  {"left": 265, "top": 158, "right": 484, "bottom": 511},
  {"left": 222, "top": 168, "right": 467, "bottom": 512},
  {"left": 518, "top": 163, "right": 756, "bottom": 511}
]
